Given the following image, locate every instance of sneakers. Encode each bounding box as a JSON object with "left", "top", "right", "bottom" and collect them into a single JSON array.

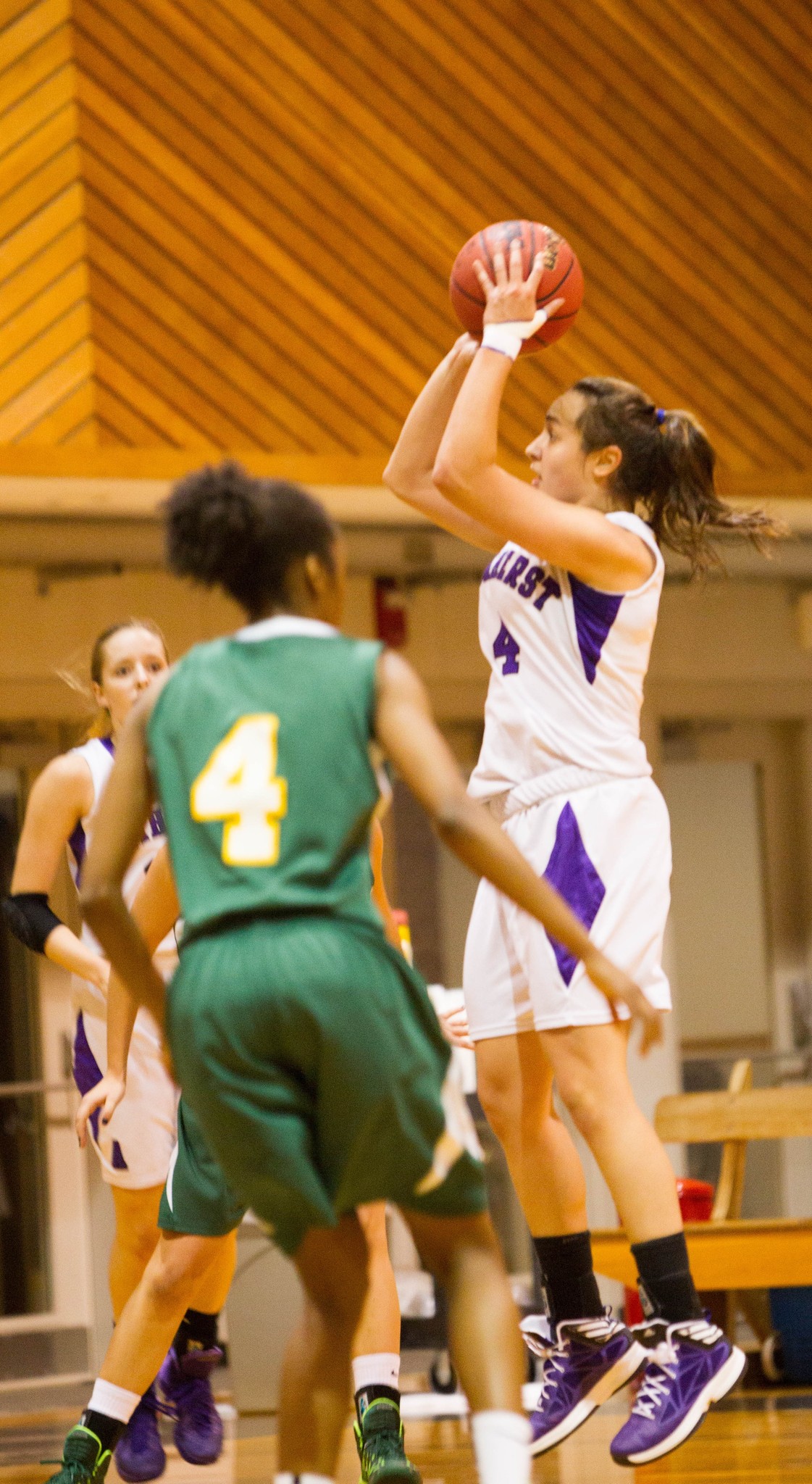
[
  {"left": 353, "top": 1396, "right": 422, "bottom": 1484},
  {"left": 40, "top": 1423, "right": 113, "bottom": 1484},
  {"left": 517, "top": 1311, "right": 647, "bottom": 1457},
  {"left": 113, "top": 1375, "right": 167, "bottom": 1483},
  {"left": 609, "top": 1318, "right": 749, "bottom": 1467},
  {"left": 157, "top": 1344, "right": 225, "bottom": 1465}
]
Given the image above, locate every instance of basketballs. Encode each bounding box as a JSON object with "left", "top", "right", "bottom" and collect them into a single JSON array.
[{"left": 448, "top": 220, "right": 588, "bottom": 352}]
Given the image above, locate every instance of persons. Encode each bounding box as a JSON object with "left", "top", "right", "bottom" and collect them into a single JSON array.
[
  {"left": 372, "top": 236, "right": 749, "bottom": 1467},
  {"left": 72, "top": 456, "right": 667, "bottom": 1483},
  {"left": 4, "top": 620, "right": 480, "bottom": 1484}
]
